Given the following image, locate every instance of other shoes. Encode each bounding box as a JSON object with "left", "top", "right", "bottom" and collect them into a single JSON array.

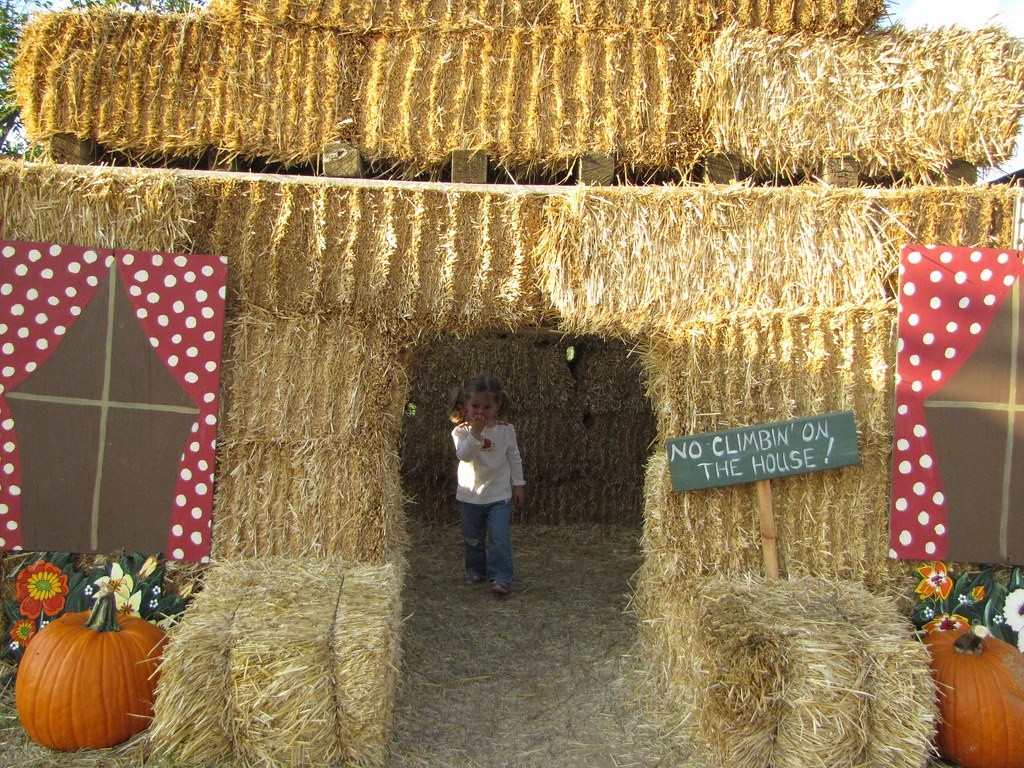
[
  {"left": 490, "top": 579, "right": 513, "bottom": 594},
  {"left": 467, "top": 574, "right": 485, "bottom": 584}
]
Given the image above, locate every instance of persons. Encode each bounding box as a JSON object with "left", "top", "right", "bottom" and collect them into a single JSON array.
[{"left": 449, "top": 373, "right": 527, "bottom": 594}]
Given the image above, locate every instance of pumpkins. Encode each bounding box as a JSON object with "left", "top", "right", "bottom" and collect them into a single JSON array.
[
  {"left": 919, "top": 625, "right": 1024, "bottom": 768},
  {"left": 15, "top": 591, "right": 169, "bottom": 752}
]
